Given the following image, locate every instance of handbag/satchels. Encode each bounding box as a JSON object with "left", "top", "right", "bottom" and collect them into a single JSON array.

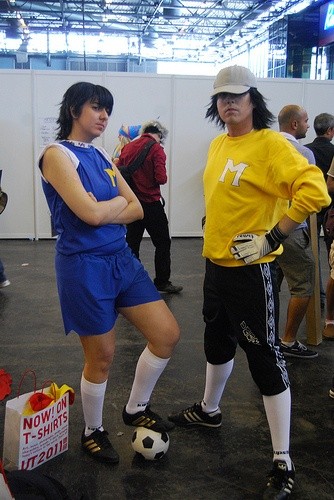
[
  {"left": 3, "top": 370, "right": 69, "bottom": 474},
  {"left": 116, "top": 140, "right": 157, "bottom": 186}
]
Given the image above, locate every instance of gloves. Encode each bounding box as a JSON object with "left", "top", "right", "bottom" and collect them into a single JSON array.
[{"left": 230, "top": 223, "right": 290, "bottom": 264}]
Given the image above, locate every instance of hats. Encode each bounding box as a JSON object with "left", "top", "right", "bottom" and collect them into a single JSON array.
[{"left": 211, "top": 65, "right": 256, "bottom": 94}]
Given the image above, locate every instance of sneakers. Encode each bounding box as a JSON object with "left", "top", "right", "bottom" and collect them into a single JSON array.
[
  {"left": 278, "top": 340, "right": 319, "bottom": 359},
  {"left": 167, "top": 402, "right": 222, "bottom": 427},
  {"left": 263, "top": 459, "right": 296, "bottom": 500},
  {"left": 81, "top": 427, "right": 119, "bottom": 465},
  {"left": 122, "top": 405, "right": 175, "bottom": 432}
]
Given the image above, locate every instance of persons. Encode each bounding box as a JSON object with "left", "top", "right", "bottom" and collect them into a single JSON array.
[
  {"left": 0, "top": 256, "right": 11, "bottom": 288},
  {"left": 114, "top": 120, "right": 184, "bottom": 295},
  {"left": 302, "top": 112, "right": 334, "bottom": 251},
  {"left": 36, "top": 82, "right": 179, "bottom": 465},
  {"left": 168, "top": 66, "right": 333, "bottom": 500},
  {"left": 323, "top": 156, "right": 333, "bottom": 342},
  {"left": 274, "top": 105, "right": 320, "bottom": 359}
]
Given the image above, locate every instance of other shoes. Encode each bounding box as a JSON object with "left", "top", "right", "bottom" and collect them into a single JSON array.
[{"left": 321, "top": 321, "right": 334, "bottom": 341}]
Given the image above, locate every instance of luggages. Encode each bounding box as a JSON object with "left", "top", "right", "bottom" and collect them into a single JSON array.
[{"left": 153, "top": 278, "right": 183, "bottom": 293}]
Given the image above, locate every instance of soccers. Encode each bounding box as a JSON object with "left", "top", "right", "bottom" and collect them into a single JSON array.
[{"left": 131, "top": 428, "right": 169, "bottom": 461}]
